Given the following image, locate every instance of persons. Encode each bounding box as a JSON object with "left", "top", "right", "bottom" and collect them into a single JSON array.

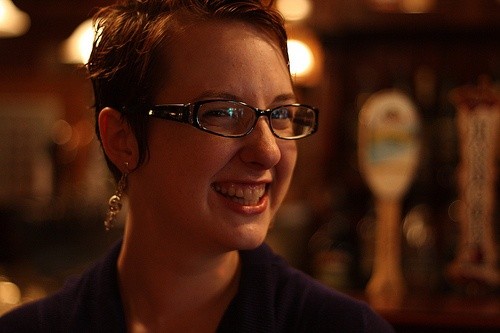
[{"left": 1, "top": 3, "right": 396, "bottom": 332}]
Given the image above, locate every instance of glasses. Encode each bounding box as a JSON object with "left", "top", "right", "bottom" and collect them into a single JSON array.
[{"left": 114, "top": 97, "right": 319, "bottom": 141}]
